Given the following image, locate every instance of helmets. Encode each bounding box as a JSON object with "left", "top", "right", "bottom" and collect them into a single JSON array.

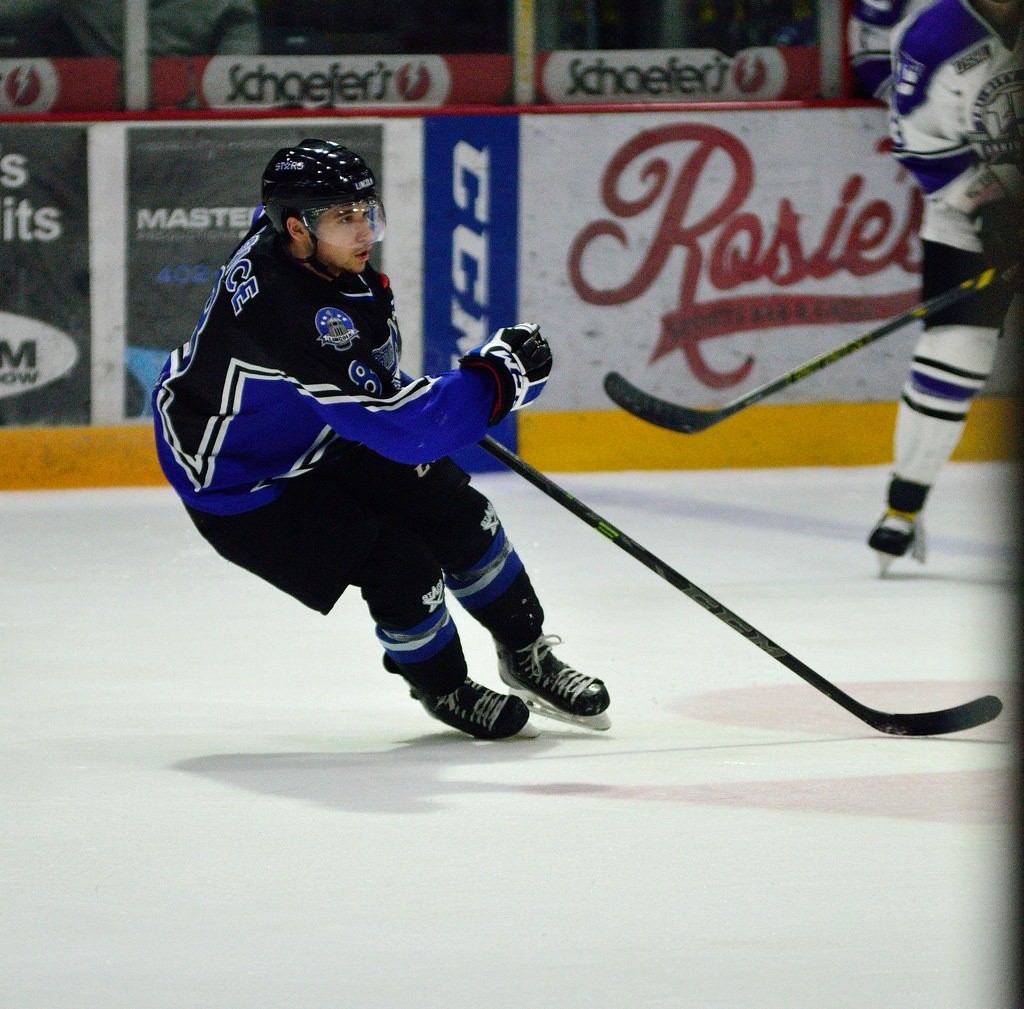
[{"left": 260, "top": 140, "right": 376, "bottom": 206}]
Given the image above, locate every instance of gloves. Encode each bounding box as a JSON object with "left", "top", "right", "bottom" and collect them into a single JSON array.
[
  {"left": 458, "top": 322, "right": 553, "bottom": 428},
  {"left": 967, "top": 198, "right": 1024, "bottom": 286}
]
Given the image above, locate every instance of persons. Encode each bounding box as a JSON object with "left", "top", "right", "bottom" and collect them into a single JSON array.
[
  {"left": 847, "top": 0, "right": 906, "bottom": 105},
  {"left": 155, "top": 140, "right": 614, "bottom": 739},
  {"left": 863, "top": 0, "right": 1024, "bottom": 574},
  {"left": 68, "top": 0, "right": 260, "bottom": 56}
]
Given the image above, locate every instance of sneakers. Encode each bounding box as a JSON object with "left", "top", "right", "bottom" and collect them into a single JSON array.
[
  {"left": 866, "top": 510, "right": 919, "bottom": 576},
  {"left": 494, "top": 634, "right": 612, "bottom": 732},
  {"left": 410, "top": 676, "right": 541, "bottom": 740}
]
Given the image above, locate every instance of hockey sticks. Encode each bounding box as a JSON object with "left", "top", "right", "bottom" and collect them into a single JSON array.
[
  {"left": 400, "top": 376, "right": 1004, "bottom": 736},
  {"left": 602, "top": 267, "right": 1012, "bottom": 436}
]
